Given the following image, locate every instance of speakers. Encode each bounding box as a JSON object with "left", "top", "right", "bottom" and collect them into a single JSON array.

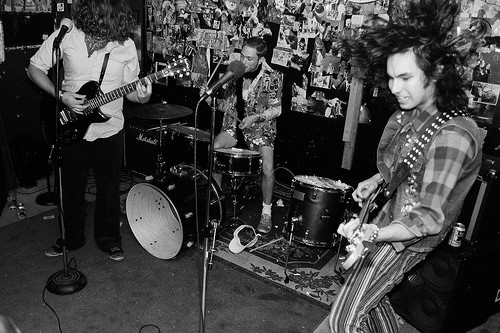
[{"left": 386, "top": 232, "right": 486, "bottom": 333}]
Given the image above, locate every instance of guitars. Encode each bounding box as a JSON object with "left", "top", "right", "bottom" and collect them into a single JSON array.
[
  {"left": 39, "top": 54, "right": 191, "bottom": 147},
  {"left": 337, "top": 185, "right": 387, "bottom": 271}
]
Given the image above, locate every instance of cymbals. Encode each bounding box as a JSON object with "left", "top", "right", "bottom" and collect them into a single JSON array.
[{"left": 127, "top": 100, "right": 193, "bottom": 121}]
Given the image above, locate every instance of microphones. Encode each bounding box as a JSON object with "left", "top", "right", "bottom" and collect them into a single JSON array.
[
  {"left": 53, "top": 18, "right": 73, "bottom": 47},
  {"left": 200, "top": 60, "right": 245, "bottom": 103}
]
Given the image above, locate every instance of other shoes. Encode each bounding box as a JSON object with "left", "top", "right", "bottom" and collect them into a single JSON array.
[
  {"left": 107, "top": 244, "right": 125, "bottom": 261},
  {"left": 44, "top": 239, "right": 68, "bottom": 257},
  {"left": 257, "top": 213, "right": 272, "bottom": 233}
]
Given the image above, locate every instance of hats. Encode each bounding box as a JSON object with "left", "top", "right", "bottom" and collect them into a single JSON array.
[{"left": 229, "top": 224, "right": 258, "bottom": 254}]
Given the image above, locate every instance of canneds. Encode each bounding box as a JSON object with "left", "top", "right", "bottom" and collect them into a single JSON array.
[
  {"left": 448, "top": 222, "right": 465, "bottom": 247},
  {"left": 7, "top": 193, "right": 17, "bottom": 209},
  {"left": 16, "top": 202, "right": 26, "bottom": 220}
]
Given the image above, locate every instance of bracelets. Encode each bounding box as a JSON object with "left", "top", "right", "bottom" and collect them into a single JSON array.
[
  {"left": 60, "top": 91, "right": 64, "bottom": 102},
  {"left": 370, "top": 227, "right": 379, "bottom": 243},
  {"left": 256, "top": 113, "right": 266, "bottom": 123}
]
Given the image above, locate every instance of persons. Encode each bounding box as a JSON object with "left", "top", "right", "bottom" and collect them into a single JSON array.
[
  {"left": 200, "top": 36, "right": 282, "bottom": 233},
  {"left": 27, "top": 0, "right": 152, "bottom": 261},
  {"left": 328, "top": 0, "right": 483, "bottom": 333}
]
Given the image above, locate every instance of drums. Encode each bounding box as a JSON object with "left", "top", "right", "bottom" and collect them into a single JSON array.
[
  {"left": 175, "top": 124, "right": 212, "bottom": 167},
  {"left": 283, "top": 174, "right": 354, "bottom": 248},
  {"left": 213, "top": 147, "right": 262, "bottom": 177},
  {"left": 126, "top": 161, "right": 227, "bottom": 260}
]
